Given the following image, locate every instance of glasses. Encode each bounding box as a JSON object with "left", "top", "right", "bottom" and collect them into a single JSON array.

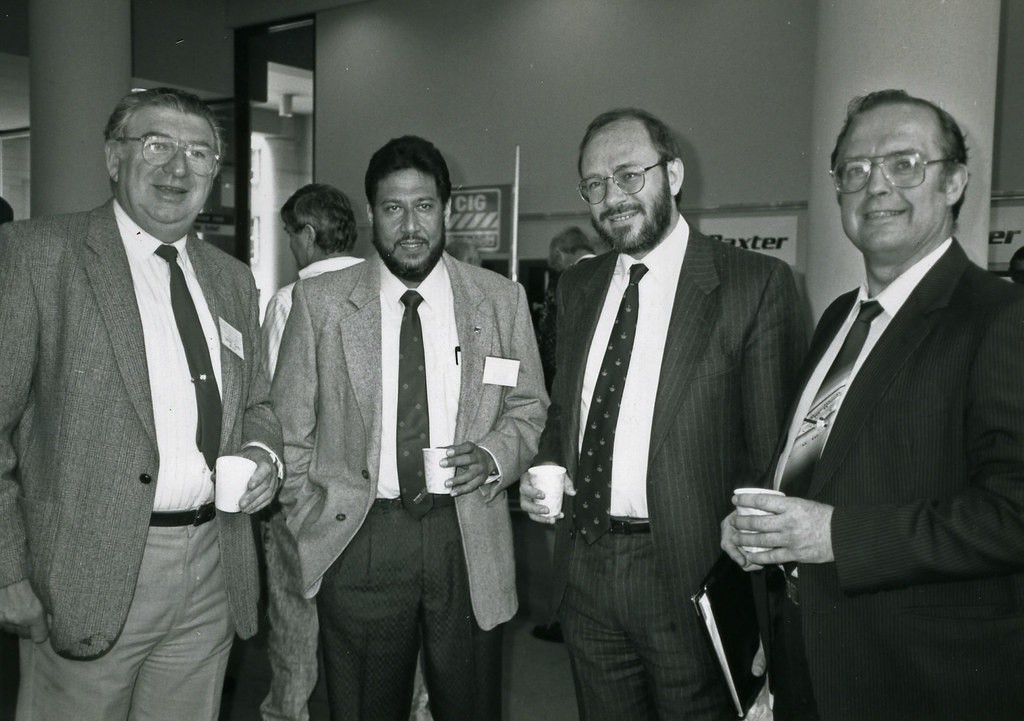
[
  {"left": 576, "top": 156, "right": 671, "bottom": 205},
  {"left": 825, "top": 152, "right": 958, "bottom": 194},
  {"left": 115, "top": 132, "right": 222, "bottom": 176}
]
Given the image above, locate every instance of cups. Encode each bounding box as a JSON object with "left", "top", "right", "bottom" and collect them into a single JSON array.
[
  {"left": 528, "top": 465, "right": 567, "bottom": 517},
  {"left": 422, "top": 448, "right": 456, "bottom": 495},
  {"left": 215, "top": 455, "right": 257, "bottom": 513},
  {"left": 734, "top": 488, "right": 786, "bottom": 553}
]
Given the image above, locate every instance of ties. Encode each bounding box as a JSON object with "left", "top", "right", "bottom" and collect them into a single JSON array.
[
  {"left": 156, "top": 244, "right": 223, "bottom": 471},
  {"left": 396, "top": 288, "right": 434, "bottom": 520},
  {"left": 778, "top": 296, "right": 884, "bottom": 575},
  {"left": 571, "top": 262, "right": 649, "bottom": 546}
]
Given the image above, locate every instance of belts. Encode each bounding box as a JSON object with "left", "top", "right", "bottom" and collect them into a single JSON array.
[
  {"left": 607, "top": 519, "right": 650, "bottom": 536},
  {"left": 148, "top": 502, "right": 217, "bottom": 528},
  {"left": 773, "top": 568, "right": 798, "bottom": 609}
]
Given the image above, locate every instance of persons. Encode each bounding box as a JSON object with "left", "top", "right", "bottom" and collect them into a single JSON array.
[
  {"left": 445, "top": 240, "right": 481, "bottom": 266},
  {"left": 540, "top": 228, "right": 597, "bottom": 374},
  {"left": 273, "top": 134, "right": 551, "bottom": 721},
  {"left": 521, "top": 110, "right": 804, "bottom": 721},
  {"left": 258, "top": 184, "right": 364, "bottom": 720},
  {"left": 718, "top": 88, "right": 1023, "bottom": 721},
  {"left": 1, "top": 86, "right": 284, "bottom": 720}
]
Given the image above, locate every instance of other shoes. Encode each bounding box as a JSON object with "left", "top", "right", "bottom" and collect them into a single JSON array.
[{"left": 532, "top": 626, "right": 566, "bottom": 644}]
